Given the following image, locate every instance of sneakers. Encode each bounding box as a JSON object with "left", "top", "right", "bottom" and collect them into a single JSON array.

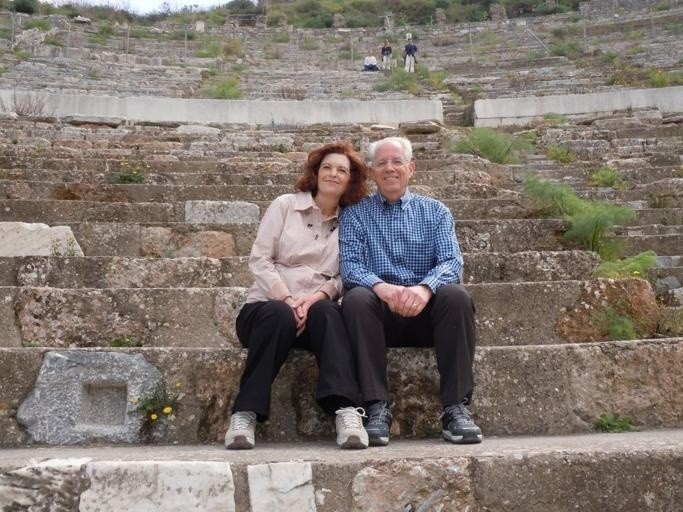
[
  {"left": 361, "top": 402, "right": 394, "bottom": 447},
  {"left": 333, "top": 404, "right": 370, "bottom": 451},
  {"left": 223, "top": 409, "right": 258, "bottom": 450},
  {"left": 439, "top": 396, "right": 484, "bottom": 445}
]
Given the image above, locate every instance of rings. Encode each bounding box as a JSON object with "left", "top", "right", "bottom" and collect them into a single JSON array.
[{"left": 414, "top": 303, "right": 417, "bottom": 306}]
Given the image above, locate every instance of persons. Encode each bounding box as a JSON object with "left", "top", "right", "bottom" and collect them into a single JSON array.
[
  {"left": 338, "top": 136, "right": 483, "bottom": 444},
  {"left": 405, "top": 37, "right": 417, "bottom": 73},
  {"left": 364, "top": 49, "right": 377, "bottom": 71},
  {"left": 382, "top": 38, "right": 392, "bottom": 71},
  {"left": 225, "top": 139, "right": 370, "bottom": 449}
]
